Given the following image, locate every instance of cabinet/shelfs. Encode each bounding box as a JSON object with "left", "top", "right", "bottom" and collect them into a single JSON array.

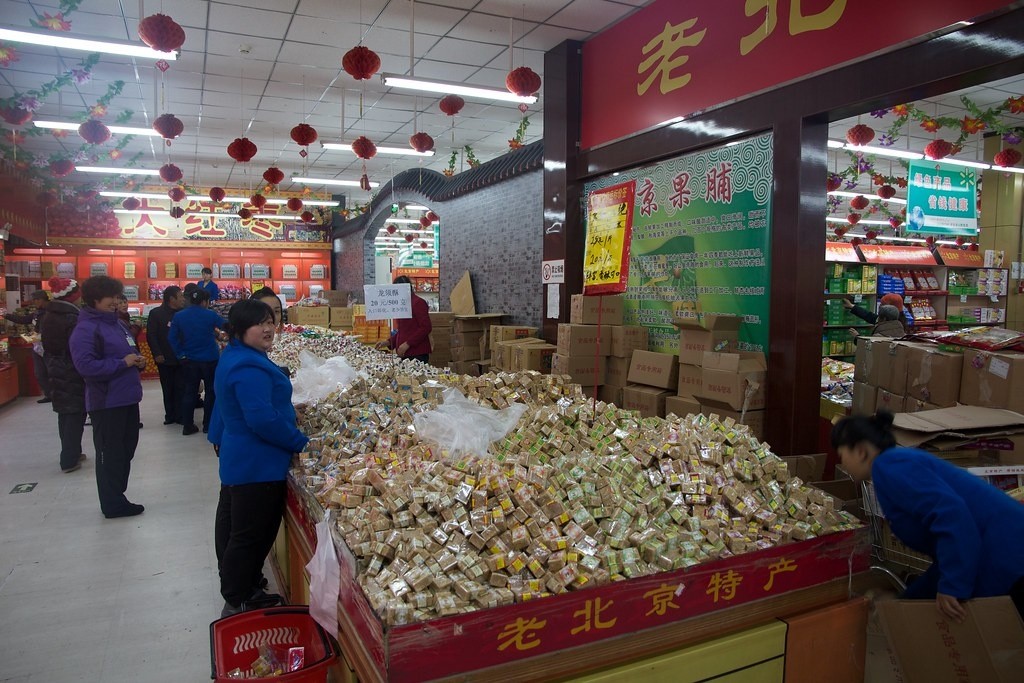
[
  {"left": 822, "top": 260, "right": 1010, "bottom": 357},
  {"left": 0, "top": 246, "right": 332, "bottom": 341}
]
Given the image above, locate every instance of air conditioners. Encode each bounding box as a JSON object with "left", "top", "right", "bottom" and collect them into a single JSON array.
[{"left": 4, "top": 274, "right": 21, "bottom": 327}]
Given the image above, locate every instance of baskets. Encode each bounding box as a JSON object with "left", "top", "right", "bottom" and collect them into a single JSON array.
[{"left": 209, "top": 605, "right": 336, "bottom": 683}]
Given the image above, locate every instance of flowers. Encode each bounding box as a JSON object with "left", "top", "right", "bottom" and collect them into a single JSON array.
[{"left": 824, "top": 94, "right": 1024, "bottom": 251}]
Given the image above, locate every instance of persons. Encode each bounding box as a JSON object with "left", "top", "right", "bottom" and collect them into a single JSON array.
[
  {"left": 147, "top": 283, "right": 230, "bottom": 435},
  {"left": 374, "top": 275, "right": 433, "bottom": 360},
  {"left": 116, "top": 295, "right": 143, "bottom": 428},
  {"left": 35, "top": 275, "right": 86, "bottom": 473},
  {"left": 849, "top": 305, "right": 904, "bottom": 346},
  {"left": 843, "top": 293, "right": 906, "bottom": 330},
  {"left": 207, "top": 286, "right": 283, "bottom": 589},
  {"left": 0, "top": 290, "right": 52, "bottom": 403},
  {"left": 69, "top": 276, "right": 146, "bottom": 518},
  {"left": 196, "top": 267, "right": 218, "bottom": 305},
  {"left": 213, "top": 300, "right": 309, "bottom": 619},
  {"left": 831, "top": 408, "right": 1024, "bottom": 624}
]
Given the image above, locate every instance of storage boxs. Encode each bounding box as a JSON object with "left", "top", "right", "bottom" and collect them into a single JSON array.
[
  {"left": 5, "top": 260, "right": 391, "bottom": 344},
  {"left": 851, "top": 333, "right": 1024, "bottom": 571},
  {"left": 823, "top": 261, "right": 1008, "bottom": 356},
  {"left": 428, "top": 295, "right": 766, "bottom": 445}
]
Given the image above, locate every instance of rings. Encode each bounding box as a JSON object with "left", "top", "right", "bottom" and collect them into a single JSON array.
[{"left": 942, "top": 601, "right": 950, "bottom": 607}]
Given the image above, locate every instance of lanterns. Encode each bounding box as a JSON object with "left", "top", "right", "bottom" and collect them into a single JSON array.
[
  {"left": 506, "top": 67, "right": 542, "bottom": 137},
  {"left": 0, "top": 13, "right": 381, "bottom": 223},
  {"left": 409, "top": 132, "right": 434, "bottom": 185},
  {"left": 439, "top": 94, "right": 465, "bottom": 143},
  {"left": 386, "top": 210, "right": 438, "bottom": 249},
  {"left": 826, "top": 124, "right": 1021, "bottom": 252}
]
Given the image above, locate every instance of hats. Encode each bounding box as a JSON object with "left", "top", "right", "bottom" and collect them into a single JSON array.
[
  {"left": 48, "top": 275, "right": 81, "bottom": 302},
  {"left": 29, "top": 290, "right": 47, "bottom": 302},
  {"left": 881, "top": 295, "right": 904, "bottom": 312}
]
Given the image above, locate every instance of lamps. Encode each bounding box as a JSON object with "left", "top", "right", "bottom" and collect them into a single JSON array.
[
  {"left": 292, "top": 71, "right": 539, "bottom": 190},
  {"left": 0, "top": 0, "right": 339, "bottom": 221}
]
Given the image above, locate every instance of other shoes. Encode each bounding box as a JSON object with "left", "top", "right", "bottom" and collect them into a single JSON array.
[
  {"left": 101, "top": 501, "right": 146, "bottom": 518},
  {"left": 203, "top": 426, "right": 210, "bottom": 433},
  {"left": 182, "top": 424, "right": 199, "bottom": 435},
  {"left": 219, "top": 592, "right": 281, "bottom": 618},
  {"left": 62, "top": 454, "right": 87, "bottom": 473},
  {"left": 37, "top": 397, "right": 51, "bottom": 403},
  {"left": 163, "top": 418, "right": 175, "bottom": 425},
  {"left": 196, "top": 400, "right": 206, "bottom": 407}
]
